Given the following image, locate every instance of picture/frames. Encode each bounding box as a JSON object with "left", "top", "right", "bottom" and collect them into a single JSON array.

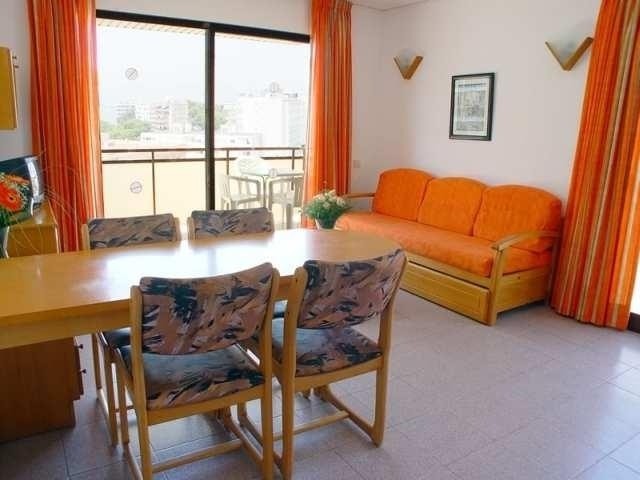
[{"left": 445, "top": 69, "right": 497, "bottom": 146}]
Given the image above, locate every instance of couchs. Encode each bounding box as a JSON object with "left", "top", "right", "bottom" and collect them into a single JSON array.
[{"left": 333, "top": 165, "right": 563, "bottom": 328}]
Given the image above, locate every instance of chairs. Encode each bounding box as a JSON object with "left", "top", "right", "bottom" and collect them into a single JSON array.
[
  {"left": 219, "top": 150, "right": 307, "bottom": 231},
  {"left": 188, "top": 209, "right": 275, "bottom": 234},
  {"left": 113, "top": 262, "right": 282, "bottom": 478},
  {"left": 282, "top": 248, "right": 408, "bottom": 475},
  {"left": 92, "top": 211, "right": 184, "bottom": 446}
]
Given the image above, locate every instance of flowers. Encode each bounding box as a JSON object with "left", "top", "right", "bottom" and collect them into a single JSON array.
[{"left": 307, "top": 189, "right": 351, "bottom": 230}]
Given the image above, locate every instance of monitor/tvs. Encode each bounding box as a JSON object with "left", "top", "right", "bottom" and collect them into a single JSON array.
[{"left": 0, "top": 154, "right": 45, "bottom": 212}]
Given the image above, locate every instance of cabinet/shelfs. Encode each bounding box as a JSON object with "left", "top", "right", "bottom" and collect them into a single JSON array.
[{"left": 1, "top": 191, "right": 90, "bottom": 448}]
